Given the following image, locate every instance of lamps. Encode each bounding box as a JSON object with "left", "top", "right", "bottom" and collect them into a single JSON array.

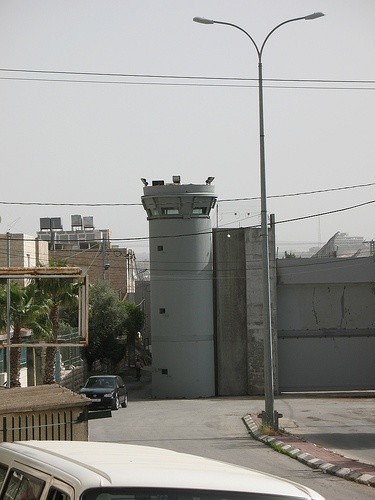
[
  {"left": 152, "top": 180, "right": 165, "bottom": 186},
  {"left": 206, "top": 177, "right": 215, "bottom": 185},
  {"left": 172, "top": 175, "right": 181, "bottom": 184},
  {"left": 141, "top": 178, "right": 148, "bottom": 186}
]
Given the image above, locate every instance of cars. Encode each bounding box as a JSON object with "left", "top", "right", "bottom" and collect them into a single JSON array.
[
  {"left": 0, "top": 437, "right": 327, "bottom": 500},
  {"left": 79, "top": 373, "right": 128, "bottom": 411}
]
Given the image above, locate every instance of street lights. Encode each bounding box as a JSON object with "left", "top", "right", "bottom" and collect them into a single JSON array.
[{"left": 193, "top": 9, "right": 330, "bottom": 433}]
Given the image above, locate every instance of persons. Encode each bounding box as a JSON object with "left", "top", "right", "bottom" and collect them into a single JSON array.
[{"left": 135, "top": 358, "right": 142, "bottom": 376}]
